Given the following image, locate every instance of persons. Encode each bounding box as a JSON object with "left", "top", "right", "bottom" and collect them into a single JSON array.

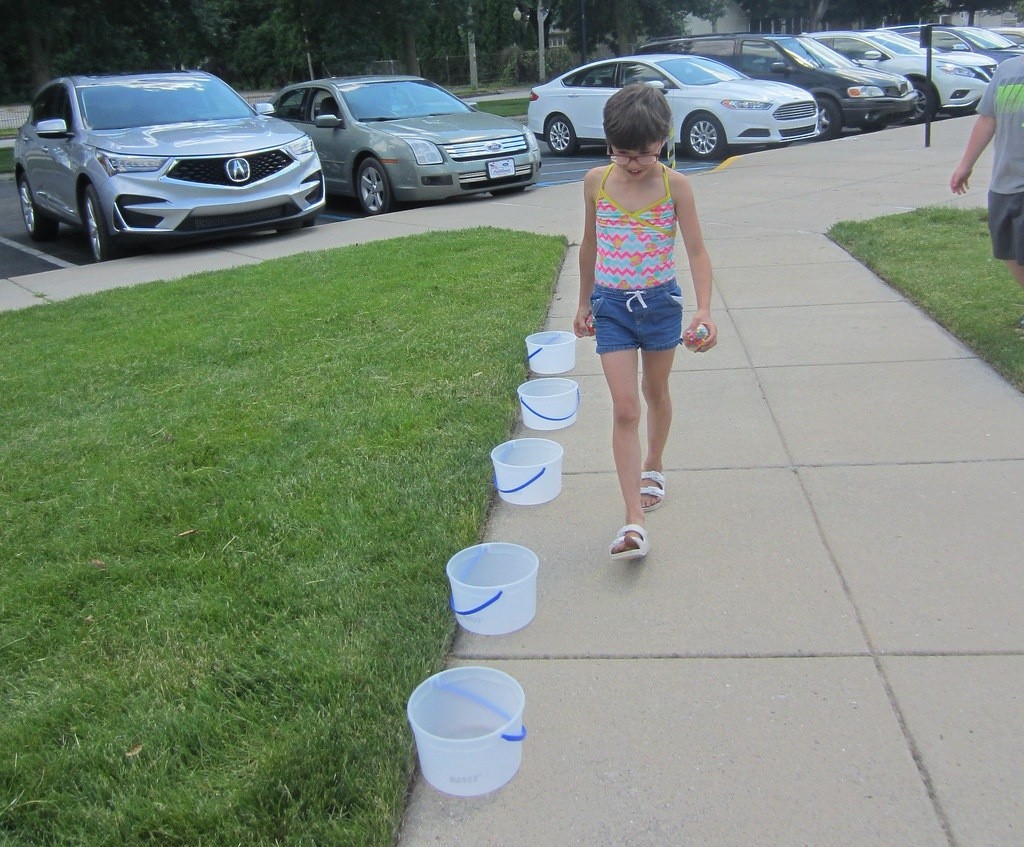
[
  {"left": 950, "top": 54, "right": 1024, "bottom": 328},
  {"left": 573, "top": 83, "right": 717, "bottom": 558}
]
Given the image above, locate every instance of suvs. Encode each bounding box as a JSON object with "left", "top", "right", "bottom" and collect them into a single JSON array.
[
  {"left": 635, "top": 31, "right": 920, "bottom": 142},
  {"left": 879, "top": 23, "right": 1024, "bottom": 67}
]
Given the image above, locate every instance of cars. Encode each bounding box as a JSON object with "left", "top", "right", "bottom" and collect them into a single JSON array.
[
  {"left": 14, "top": 70, "right": 325, "bottom": 263},
  {"left": 268, "top": 75, "right": 541, "bottom": 216},
  {"left": 528, "top": 54, "right": 819, "bottom": 160},
  {"left": 801, "top": 30, "right": 998, "bottom": 125},
  {"left": 987, "top": 27, "right": 1024, "bottom": 46}
]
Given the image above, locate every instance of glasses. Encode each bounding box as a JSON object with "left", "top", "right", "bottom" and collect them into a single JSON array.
[{"left": 606, "top": 134, "right": 663, "bottom": 167}]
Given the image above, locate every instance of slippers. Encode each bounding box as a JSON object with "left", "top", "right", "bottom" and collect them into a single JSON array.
[
  {"left": 608, "top": 524, "right": 649, "bottom": 562},
  {"left": 639, "top": 469, "right": 666, "bottom": 513}
]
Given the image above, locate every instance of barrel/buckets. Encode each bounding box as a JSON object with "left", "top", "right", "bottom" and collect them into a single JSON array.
[
  {"left": 446, "top": 542, "right": 540, "bottom": 636},
  {"left": 525, "top": 331, "right": 577, "bottom": 374},
  {"left": 491, "top": 437, "right": 564, "bottom": 505},
  {"left": 406, "top": 665, "right": 527, "bottom": 797},
  {"left": 517, "top": 377, "right": 580, "bottom": 430}
]
